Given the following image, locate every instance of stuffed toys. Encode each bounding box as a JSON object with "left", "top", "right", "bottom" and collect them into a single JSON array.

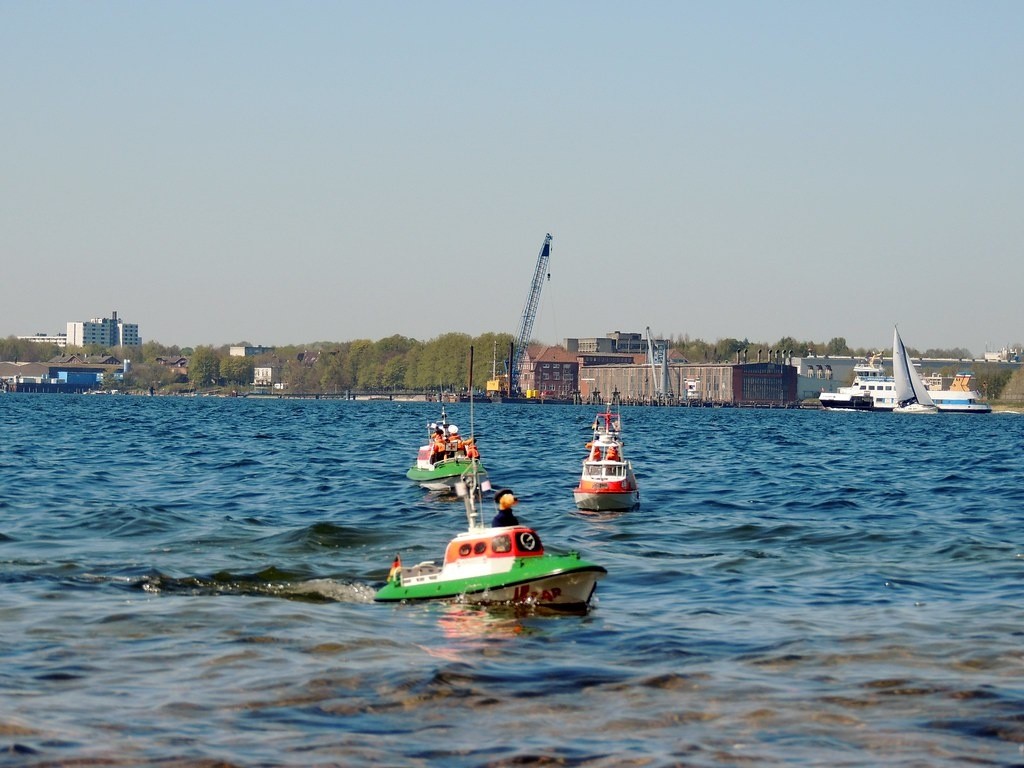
[
  {"left": 491, "top": 489, "right": 519, "bottom": 527},
  {"left": 463, "top": 437, "right": 480, "bottom": 462},
  {"left": 430, "top": 424, "right": 464, "bottom": 464},
  {"left": 606, "top": 446, "right": 619, "bottom": 461},
  {"left": 585, "top": 436, "right": 600, "bottom": 461}
]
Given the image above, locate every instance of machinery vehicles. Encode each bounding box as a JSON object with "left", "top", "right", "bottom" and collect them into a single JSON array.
[{"left": 487, "top": 232, "right": 555, "bottom": 397}]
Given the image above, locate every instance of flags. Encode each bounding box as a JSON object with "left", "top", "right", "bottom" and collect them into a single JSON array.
[{"left": 387, "top": 554, "right": 400, "bottom": 581}]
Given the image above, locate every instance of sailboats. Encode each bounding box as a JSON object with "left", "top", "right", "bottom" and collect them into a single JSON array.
[{"left": 891, "top": 324, "right": 939, "bottom": 414}]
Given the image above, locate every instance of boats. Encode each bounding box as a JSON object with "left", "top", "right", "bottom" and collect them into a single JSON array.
[
  {"left": 408, "top": 400, "right": 494, "bottom": 499},
  {"left": 818, "top": 350, "right": 994, "bottom": 415},
  {"left": 576, "top": 401, "right": 654, "bottom": 518},
  {"left": 372, "top": 451, "right": 624, "bottom": 618}
]
